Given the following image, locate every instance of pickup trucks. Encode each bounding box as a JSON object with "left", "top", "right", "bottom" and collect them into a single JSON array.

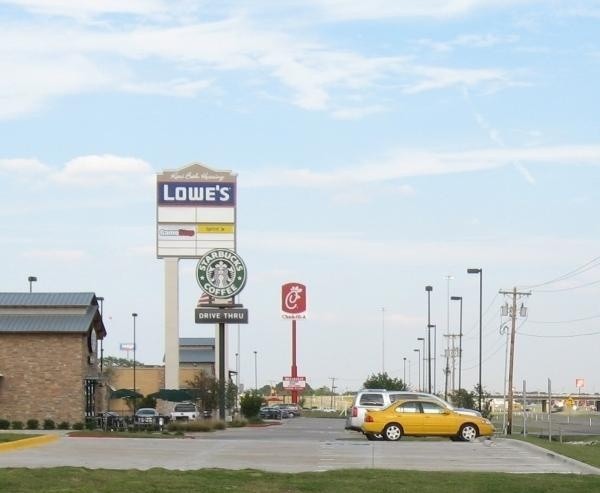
[{"left": 169, "top": 402, "right": 201, "bottom": 422}]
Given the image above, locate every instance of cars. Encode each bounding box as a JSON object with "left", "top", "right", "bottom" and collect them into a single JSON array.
[
  {"left": 133, "top": 408, "right": 160, "bottom": 427},
  {"left": 361, "top": 398, "right": 496, "bottom": 441},
  {"left": 259, "top": 403, "right": 335, "bottom": 420}
]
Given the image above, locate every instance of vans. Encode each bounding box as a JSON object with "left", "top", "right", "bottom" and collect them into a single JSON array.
[{"left": 344, "top": 388, "right": 483, "bottom": 441}]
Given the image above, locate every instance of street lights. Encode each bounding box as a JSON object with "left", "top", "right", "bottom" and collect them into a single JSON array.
[
  {"left": 28, "top": 276, "right": 37, "bottom": 293},
  {"left": 236, "top": 351, "right": 257, "bottom": 412},
  {"left": 132, "top": 313, "right": 138, "bottom": 417},
  {"left": 403, "top": 269, "right": 482, "bottom": 412},
  {"left": 97, "top": 297, "right": 104, "bottom": 373}
]
